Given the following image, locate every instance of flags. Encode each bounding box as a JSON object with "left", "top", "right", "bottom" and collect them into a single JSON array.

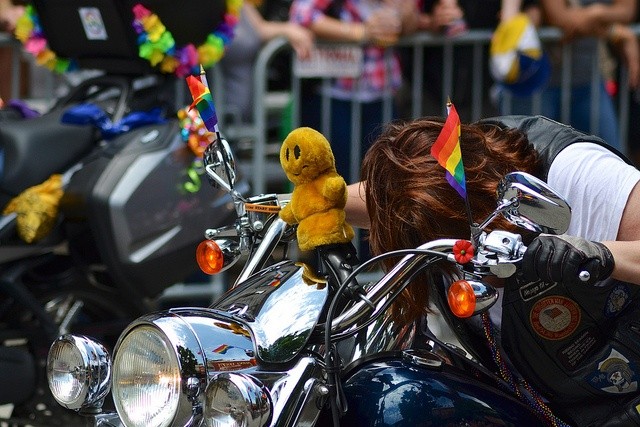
[
  {"left": 431, "top": 104, "right": 469, "bottom": 199},
  {"left": 185, "top": 74, "right": 218, "bottom": 133}
]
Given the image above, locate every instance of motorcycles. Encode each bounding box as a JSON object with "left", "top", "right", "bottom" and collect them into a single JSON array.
[
  {"left": 46, "top": 135, "right": 640, "bottom": 427},
  {"left": 1, "top": 68, "right": 236, "bottom": 426}
]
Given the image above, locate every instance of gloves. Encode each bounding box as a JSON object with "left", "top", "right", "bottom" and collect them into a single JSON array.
[{"left": 522, "top": 232, "right": 615, "bottom": 286}]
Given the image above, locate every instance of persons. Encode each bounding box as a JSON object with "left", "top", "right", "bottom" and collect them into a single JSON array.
[
  {"left": 288, "top": 0, "right": 465, "bottom": 185},
  {"left": 498, "top": 0, "right": 522, "bottom": 22},
  {"left": 236, "top": 113, "right": 640, "bottom": 426},
  {"left": 489, "top": 0, "right": 556, "bottom": 118},
  {"left": 540, "top": 0, "right": 640, "bottom": 158},
  {"left": 208, "top": 1, "right": 317, "bottom": 127},
  {"left": 431, "top": 0, "right": 464, "bottom": 35}
]
